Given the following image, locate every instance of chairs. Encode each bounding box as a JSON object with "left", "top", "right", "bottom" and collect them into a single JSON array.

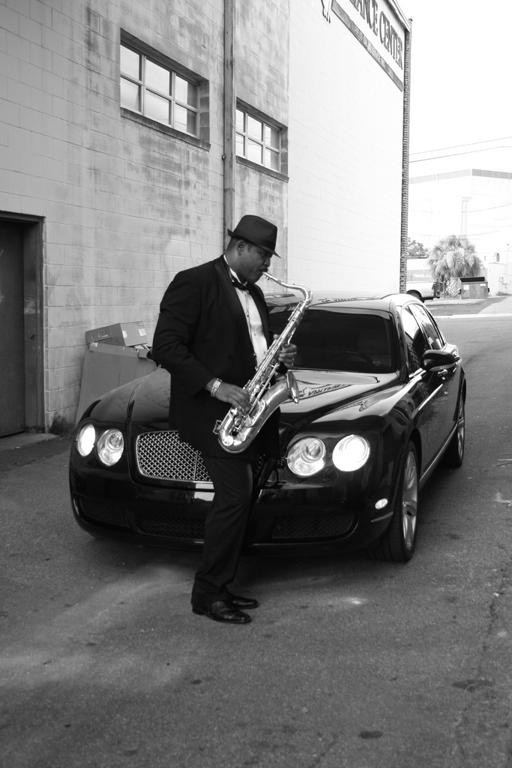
[{"left": 358, "top": 324, "right": 393, "bottom": 366}]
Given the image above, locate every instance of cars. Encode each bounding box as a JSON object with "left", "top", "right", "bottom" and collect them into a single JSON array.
[{"left": 65, "top": 292, "right": 468, "bottom": 564}]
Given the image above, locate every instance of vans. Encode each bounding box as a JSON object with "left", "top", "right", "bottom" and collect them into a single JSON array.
[{"left": 406, "top": 257, "right": 435, "bottom": 303}]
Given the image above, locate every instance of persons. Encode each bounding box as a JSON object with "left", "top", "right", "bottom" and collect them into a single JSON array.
[{"left": 151, "top": 213, "right": 298, "bottom": 625}]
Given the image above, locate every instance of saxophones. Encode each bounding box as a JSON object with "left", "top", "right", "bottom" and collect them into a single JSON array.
[{"left": 213, "top": 272, "right": 316, "bottom": 456}]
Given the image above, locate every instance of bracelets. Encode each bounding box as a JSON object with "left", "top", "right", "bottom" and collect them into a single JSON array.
[{"left": 210, "top": 378, "right": 222, "bottom": 398}]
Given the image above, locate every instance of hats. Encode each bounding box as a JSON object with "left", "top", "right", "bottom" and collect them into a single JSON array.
[{"left": 228, "top": 214, "right": 282, "bottom": 259}]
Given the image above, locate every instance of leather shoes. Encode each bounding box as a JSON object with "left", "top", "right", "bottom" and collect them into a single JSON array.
[{"left": 192, "top": 585, "right": 260, "bottom": 626}]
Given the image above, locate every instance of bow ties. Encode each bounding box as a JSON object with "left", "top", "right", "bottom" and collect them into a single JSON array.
[{"left": 231, "top": 277, "right": 256, "bottom": 294}]
